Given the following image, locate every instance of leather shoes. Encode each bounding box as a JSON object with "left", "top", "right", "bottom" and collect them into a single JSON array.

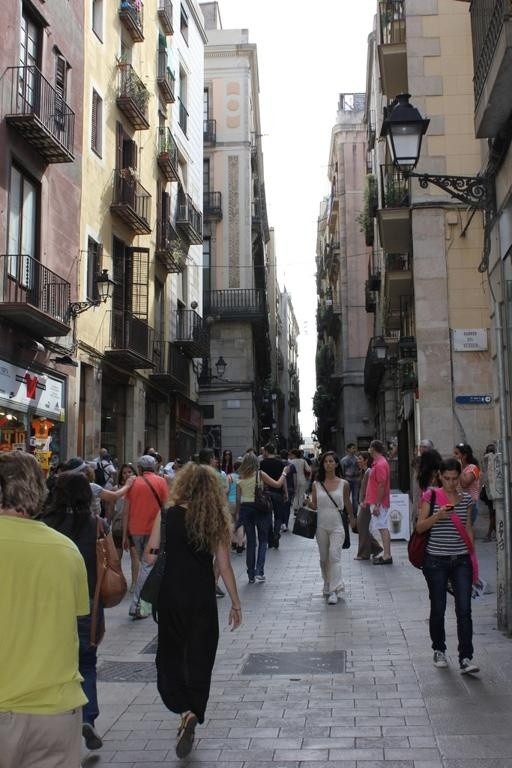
[
  {"left": 373, "top": 556, "right": 392, "bottom": 565},
  {"left": 237, "top": 544, "right": 244, "bottom": 553}
]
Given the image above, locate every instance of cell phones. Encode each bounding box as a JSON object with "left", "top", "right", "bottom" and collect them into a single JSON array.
[{"left": 446, "top": 506, "right": 454, "bottom": 511}]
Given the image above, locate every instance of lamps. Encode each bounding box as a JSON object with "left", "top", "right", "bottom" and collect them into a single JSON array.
[
  {"left": 196, "top": 352, "right": 230, "bottom": 384},
  {"left": 370, "top": 331, "right": 401, "bottom": 377},
  {"left": 376, "top": 86, "right": 498, "bottom": 219},
  {"left": 66, "top": 265, "right": 116, "bottom": 320}
]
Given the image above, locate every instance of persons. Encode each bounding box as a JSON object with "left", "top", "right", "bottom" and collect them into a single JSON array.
[
  {"left": 308, "top": 440, "right": 498, "bottom": 608},
  {"left": 416, "top": 457, "right": 482, "bottom": 675},
  {"left": 1, "top": 441, "right": 312, "bottom": 768}
]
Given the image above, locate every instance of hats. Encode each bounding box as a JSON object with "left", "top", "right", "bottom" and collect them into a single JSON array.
[
  {"left": 136, "top": 454, "right": 156, "bottom": 468},
  {"left": 65, "top": 457, "right": 84, "bottom": 472}
]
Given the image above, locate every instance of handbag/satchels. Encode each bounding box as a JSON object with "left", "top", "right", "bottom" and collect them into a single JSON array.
[
  {"left": 292, "top": 502, "right": 318, "bottom": 539},
  {"left": 407, "top": 486, "right": 435, "bottom": 568},
  {"left": 96, "top": 514, "right": 127, "bottom": 608},
  {"left": 340, "top": 511, "right": 350, "bottom": 549},
  {"left": 139, "top": 506, "right": 168, "bottom": 605},
  {"left": 255, "top": 469, "right": 273, "bottom": 512}
]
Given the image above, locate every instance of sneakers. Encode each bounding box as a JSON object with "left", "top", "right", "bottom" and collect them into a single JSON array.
[
  {"left": 433, "top": 650, "right": 448, "bottom": 668},
  {"left": 328, "top": 591, "right": 337, "bottom": 604},
  {"left": 175, "top": 709, "right": 198, "bottom": 758},
  {"left": 459, "top": 657, "right": 480, "bottom": 675},
  {"left": 280, "top": 523, "right": 287, "bottom": 532},
  {"left": 129, "top": 603, "right": 137, "bottom": 616},
  {"left": 323, "top": 588, "right": 329, "bottom": 595},
  {"left": 83, "top": 722, "right": 103, "bottom": 750},
  {"left": 255, "top": 575, "right": 265, "bottom": 582}
]
